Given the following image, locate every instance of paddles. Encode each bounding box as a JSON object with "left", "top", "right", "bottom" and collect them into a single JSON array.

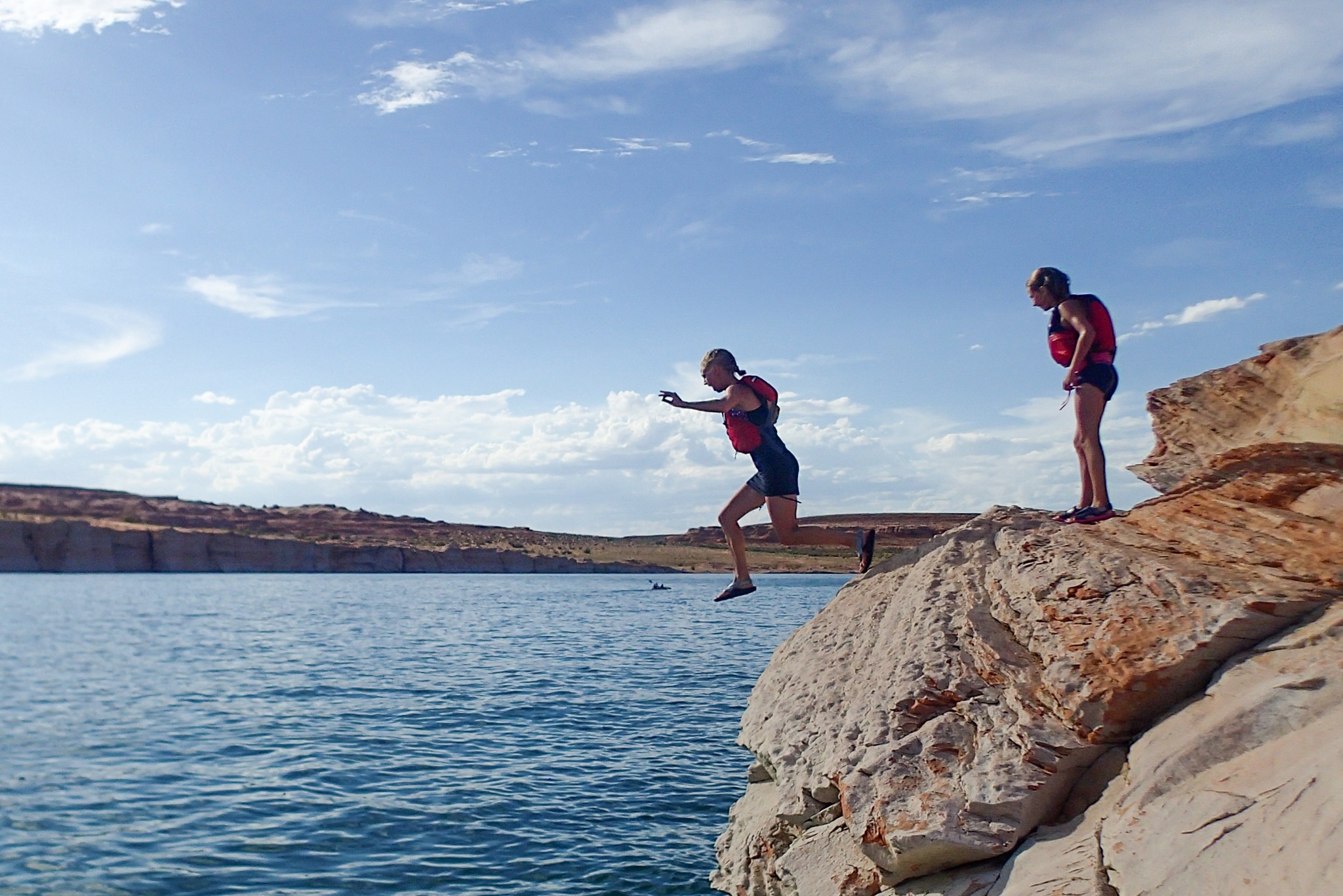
[{"left": 648, "top": 580, "right": 658, "bottom": 587}]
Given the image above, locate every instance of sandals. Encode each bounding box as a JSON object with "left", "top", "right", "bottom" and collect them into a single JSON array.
[
  {"left": 1066, "top": 503, "right": 1115, "bottom": 524},
  {"left": 855, "top": 529, "right": 875, "bottom": 573},
  {"left": 1052, "top": 506, "right": 1083, "bottom": 521},
  {"left": 714, "top": 578, "right": 756, "bottom": 601}
]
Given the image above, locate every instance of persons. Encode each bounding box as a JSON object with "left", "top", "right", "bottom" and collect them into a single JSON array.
[
  {"left": 658, "top": 348, "right": 875, "bottom": 602},
  {"left": 1025, "top": 266, "right": 1119, "bottom": 523}
]
